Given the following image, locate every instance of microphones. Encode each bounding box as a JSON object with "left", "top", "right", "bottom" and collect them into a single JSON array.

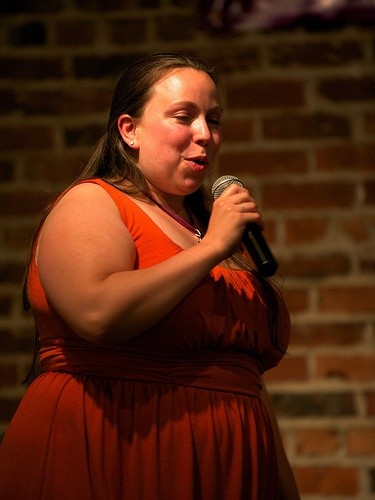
[{"left": 212, "top": 175, "right": 278, "bottom": 277}]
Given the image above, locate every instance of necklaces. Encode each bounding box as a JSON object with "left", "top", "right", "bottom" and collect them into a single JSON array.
[{"left": 117, "top": 170, "right": 204, "bottom": 245}]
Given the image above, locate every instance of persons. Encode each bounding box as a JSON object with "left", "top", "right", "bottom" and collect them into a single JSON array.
[{"left": 0, "top": 54, "right": 300, "bottom": 500}]
